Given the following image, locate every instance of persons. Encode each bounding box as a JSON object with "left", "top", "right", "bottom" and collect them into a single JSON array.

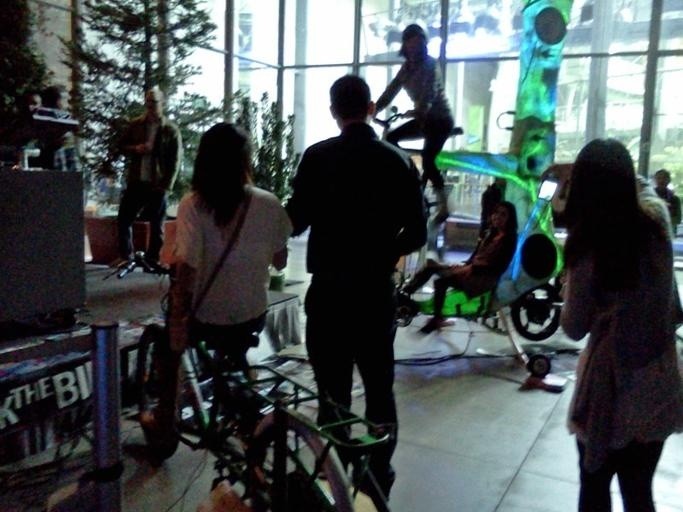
[
  {"left": 635, "top": 174, "right": 683, "bottom": 324},
  {"left": 281, "top": 74, "right": 430, "bottom": 503},
  {"left": 5, "top": 93, "right": 41, "bottom": 143},
  {"left": 557, "top": 136, "right": 683, "bottom": 512},
  {"left": 368, "top": 21, "right": 454, "bottom": 230},
  {"left": 401, "top": 196, "right": 518, "bottom": 334},
  {"left": 136, "top": 120, "right": 293, "bottom": 438},
  {"left": 653, "top": 168, "right": 682, "bottom": 237},
  {"left": 41, "top": 84, "right": 82, "bottom": 173},
  {"left": 110, "top": 87, "right": 182, "bottom": 273}
]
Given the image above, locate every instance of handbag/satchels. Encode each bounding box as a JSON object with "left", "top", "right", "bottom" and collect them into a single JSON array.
[
  {"left": 673, "top": 280, "right": 682, "bottom": 329},
  {"left": 159, "top": 290, "right": 199, "bottom": 348}
]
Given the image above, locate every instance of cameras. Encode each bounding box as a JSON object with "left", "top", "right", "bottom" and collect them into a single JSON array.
[{"left": 539, "top": 176, "right": 561, "bottom": 203}]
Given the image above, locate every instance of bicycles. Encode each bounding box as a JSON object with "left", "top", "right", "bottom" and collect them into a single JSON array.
[
  {"left": 108, "top": 247, "right": 394, "bottom": 511},
  {"left": 368, "top": 106, "right": 462, "bottom": 225}
]
